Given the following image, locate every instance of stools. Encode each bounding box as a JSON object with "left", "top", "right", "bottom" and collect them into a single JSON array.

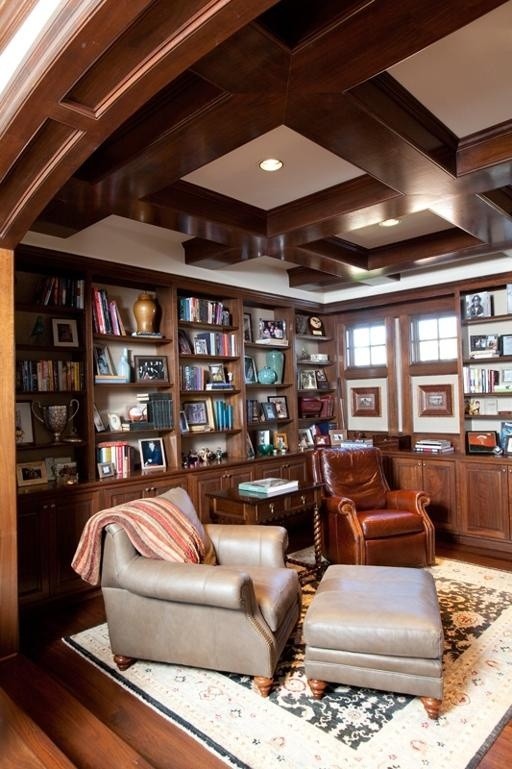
[{"left": 303, "top": 562, "right": 446, "bottom": 722}]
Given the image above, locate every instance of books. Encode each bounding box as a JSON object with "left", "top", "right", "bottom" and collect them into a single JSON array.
[
  {"left": 463, "top": 367, "right": 500, "bottom": 394},
  {"left": 89, "top": 286, "right": 127, "bottom": 337},
  {"left": 252, "top": 400, "right": 258, "bottom": 425},
  {"left": 470, "top": 350, "right": 497, "bottom": 354},
  {"left": 13, "top": 274, "right": 84, "bottom": 310},
  {"left": 473, "top": 354, "right": 501, "bottom": 359},
  {"left": 413, "top": 439, "right": 454, "bottom": 453},
  {"left": 239, "top": 486, "right": 298, "bottom": 499},
  {"left": 341, "top": 442, "right": 373, "bottom": 449},
  {"left": 15, "top": 360, "right": 86, "bottom": 392},
  {"left": 121, "top": 392, "right": 173, "bottom": 432},
  {"left": 246, "top": 399, "right": 252, "bottom": 426},
  {"left": 95, "top": 375, "right": 127, "bottom": 380},
  {"left": 95, "top": 379, "right": 126, "bottom": 384},
  {"left": 238, "top": 477, "right": 299, "bottom": 493},
  {"left": 178, "top": 296, "right": 237, "bottom": 432},
  {"left": 96, "top": 440, "right": 135, "bottom": 474},
  {"left": 321, "top": 394, "right": 334, "bottom": 417}
]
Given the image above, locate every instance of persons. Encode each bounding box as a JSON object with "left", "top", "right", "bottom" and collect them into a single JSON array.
[
  {"left": 316, "top": 436, "right": 327, "bottom": 445},
  {"left": 260, "top": 322, "right": 283, "bottom": 339},
  {"left": 316, "top": 371, "right": 325, "bottom": 381},
  {"left": 303, "top": 376, "right": 308, "bottom": 387},
  {"left": 475, "top": 336, "right": 487, "bottom": 348},
  {"left": 469, "top": 294, "right": 484, "bottom": 320},
  {"left": 464, "top": 400, "right": 472, "bottom": 414},
  {"left": 138, "top": 359, "right": 166, "bottom": 381},
  {"left": 469, "top": 401, "right": 480, "bottom": 415},
  {"left": 308, "top": 371, "right": 314, "bottom": 388},
  {"left": 276, "top": 436, "right": 284, "bottom": 448},
  {"left": 144, "top": 441, "right": 163, "bottom": 466},
  {"left": 275, "top": 400, "right": 282, "bottom": 415},
  {"left": 28, "top": 468, "right": 41, "bottom": 479},
  {"left": 472, "top": 407, "right": 479, "bottom": 415}
]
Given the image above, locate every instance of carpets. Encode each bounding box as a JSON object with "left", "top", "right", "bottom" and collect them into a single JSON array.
[{"left": 58, "top": 550, "right": 512, "bottom": 768}]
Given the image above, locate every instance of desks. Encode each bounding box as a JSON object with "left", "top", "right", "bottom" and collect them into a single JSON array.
[{"left": 204, "top": 483, "right": 326, "bottom": 570}]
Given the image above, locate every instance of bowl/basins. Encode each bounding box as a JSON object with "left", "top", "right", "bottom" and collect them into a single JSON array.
[{"left": 258, "top": 444, "right": 273, "bottom": 456}]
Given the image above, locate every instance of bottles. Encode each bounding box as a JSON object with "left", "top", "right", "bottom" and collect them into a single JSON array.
[
  {"left": 132, "top": 288, "right": 159, "bottom": 335},
  {"left": 258, "top": 366, "right": 276, "bottom": 385}
]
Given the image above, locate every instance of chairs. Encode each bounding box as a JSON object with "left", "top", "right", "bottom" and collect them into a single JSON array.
[
  {"left": 72, "top": 487, "right": 300, "bottom": 698},
  {"left": 307, "top": 444, "right": 439, "bottom": 573}
]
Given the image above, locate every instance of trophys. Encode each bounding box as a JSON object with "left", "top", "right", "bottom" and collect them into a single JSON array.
[{"left": 31, "top": 398, "right": 79, "bottom": 446}]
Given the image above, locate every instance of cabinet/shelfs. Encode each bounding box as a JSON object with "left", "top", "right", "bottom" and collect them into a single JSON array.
[
  {"left": 92, "top": 262, "right": 182, "bottom": 483},
  {"left": 239, "top": 286, "right": 297, "bottom": 462},
  {"left": 16, "top": 254, "right": 98, "bottom": 489},
  {"left": 255, "top": 452, "right": 316, "bottom": 523},
  {"left": 393, "top": 454, "right": 457, "bottom": 536},
  {"left": 291, "top": 296, "right": 348, "bottom": 451},
  {"left": 17, "top": 483, "right": 105, "bottom": 610},
  {"left": 456, "top": 457, "right": 512, "bottom": 553},
  {"left": 173, "top": 274, "right": 243, "bottom": 465},
  {"left": 104, "top": 472, "right": 193, "bottom": 513},
  {"left": 457, "top": 273, "right": 511, "bottom": 460},
  {"left": 189, "top": 461, "right": 256, "bottom": 523}
]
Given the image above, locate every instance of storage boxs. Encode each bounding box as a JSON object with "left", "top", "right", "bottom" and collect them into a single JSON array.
[{"left": 297, "top": 395, "right": 322, "bottom": 419}]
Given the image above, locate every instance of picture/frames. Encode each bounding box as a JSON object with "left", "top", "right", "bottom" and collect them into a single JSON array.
[
  {"left": 93, "top": 402, "right": 107, "bottom": 433},
  {"left": 260, "top": 402, "right": 278, "bottom": 421},
  {"left": 465, "top": 429, "right": 499, "bottom": 456},
  {"left": 242, "top": 355, "right": 259, "bottom": 386},
  {"left": 328, "top": 428, "right": 347, "bottom": 448},
  {"left": 93, "top": 341, "right": 115, "bottom": 377},
  {"left": 16, "top": 400, "right": 37, "bottom": 446},
  {"left": 51, "top": 316, "right": 81, "bottom": 349},
  {"left": 298, "top": 426, "right": 316, "bottom": 449},
  {"left": 417, "top": 383, "right": 454, "bottom": 418},
  {"left": 97, "top": 461, "right": 116, "bottom": 479},
  {"left": 134, "top": 354, "right": 170, "bottom": 384},
  {"left": 207, "top": 363, "right": 226, "bottom": 386},
  {"left": 244, "top": 310, "right": 253, "bottom": 344},
  {"left": 351, "top": 386, "right": 381, "bottom": 417},
  {"left": 18, "top": 460, "right": 48, "bottom": 486},
  {"left": 272, "top": 432, "right": 288, "bottom": 449},
  {"left": 193, "top": 335, "right": 210, "bottom": 356},
  {"left": 106, "top": 412, "right": 124, "bottom": 433},
  {"left": 179, "top": 410, "right": 190, "bottom": 433},
  {"left": 138, "top": 436, "right": 166, "bottom": 472},
  {"left": 266, "top": 394, "right": 288, "bottom": 420},
  {"left": 502, "top": 433, "right": 512, "bottom": 456},
  {"left": 181, "top": 393, "right": 215, "bottom": 432}
]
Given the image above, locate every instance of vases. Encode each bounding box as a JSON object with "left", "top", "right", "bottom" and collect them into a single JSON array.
[
  {"left": 257, "top": 363, "right": 277, "bottom": 384},
  {"left": 133, "top": 293, "right": 157, "bottom": 334}
]
